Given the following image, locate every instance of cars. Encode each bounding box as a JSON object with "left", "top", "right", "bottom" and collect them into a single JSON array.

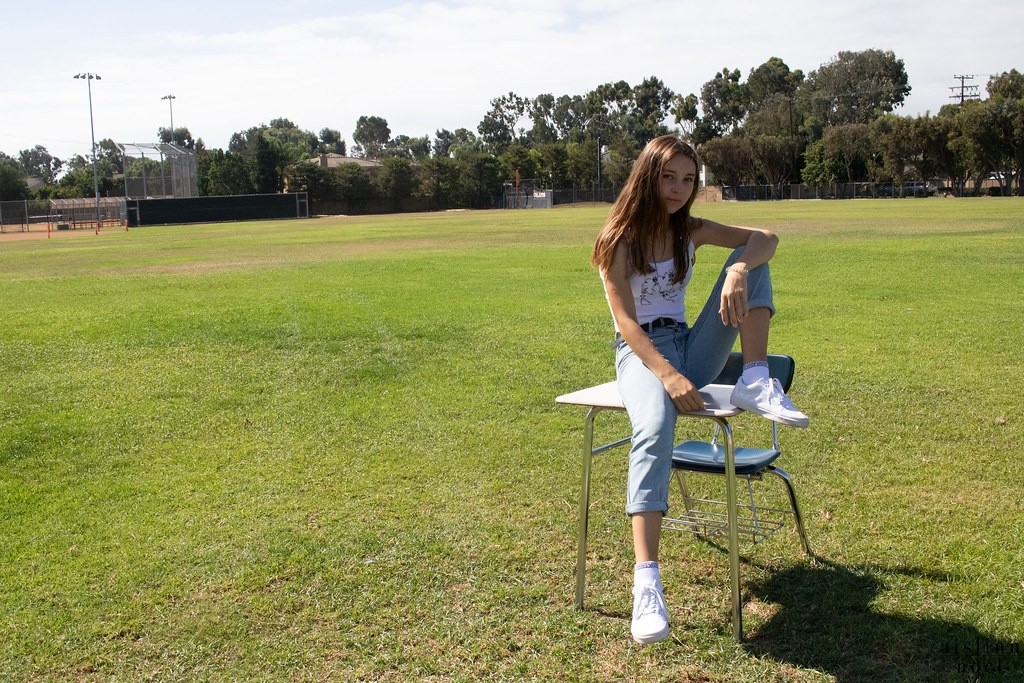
[{"left": 904, "top": 181, "right": 924, "bottom": 196}]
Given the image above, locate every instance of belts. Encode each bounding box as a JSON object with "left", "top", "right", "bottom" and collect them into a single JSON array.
[{"left": 610, "top": 316, "right": 682, "bottom": 349}]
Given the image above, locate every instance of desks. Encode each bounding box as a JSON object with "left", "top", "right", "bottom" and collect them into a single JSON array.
[{"left": 555, "top": 379, "right": 749, "bottom": 643}]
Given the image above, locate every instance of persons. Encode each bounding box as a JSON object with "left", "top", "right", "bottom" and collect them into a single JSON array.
[{"left": 591, "top": 134, "right": 809, "bottom": 643}]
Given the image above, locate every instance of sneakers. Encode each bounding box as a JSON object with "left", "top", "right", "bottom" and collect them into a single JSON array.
[
  {"left": 629, "top": 579, "right": 671, "bottom": 645},
  {"left": 729, "top": 375, "right": 810, "bottom": 429}
]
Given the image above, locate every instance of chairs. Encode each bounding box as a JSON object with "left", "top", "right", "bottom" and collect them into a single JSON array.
[{"left": 660, "top": 352, "right": 814, "bottom": 558}]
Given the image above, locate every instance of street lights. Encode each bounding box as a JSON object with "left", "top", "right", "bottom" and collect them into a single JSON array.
[
  {"left": 161, "top": 94, "right": 176, "bottom": 145},
  {"left": 73, "top": 72, "right": 102, "bottom": 227}
]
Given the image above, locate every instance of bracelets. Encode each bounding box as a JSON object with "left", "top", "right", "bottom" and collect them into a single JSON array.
[{"left": 725, "top": 265, "right": 750, "bottom": 278}]
